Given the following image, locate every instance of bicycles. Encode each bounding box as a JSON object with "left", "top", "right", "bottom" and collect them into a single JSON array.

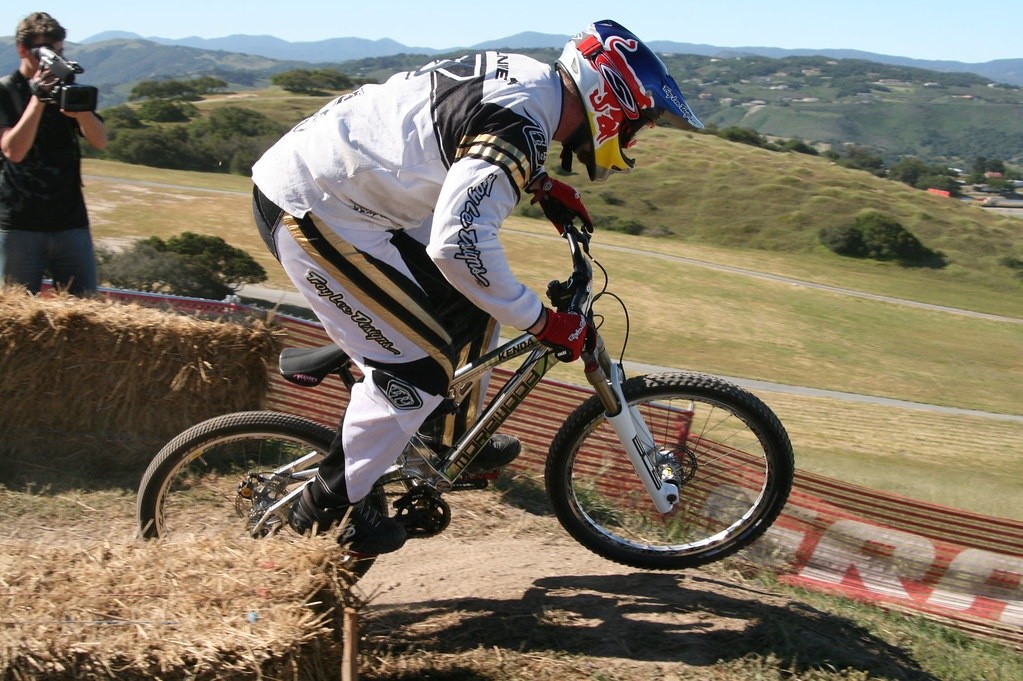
[{"left": 134, "top": 218, "right": 801, "bottom": 590}]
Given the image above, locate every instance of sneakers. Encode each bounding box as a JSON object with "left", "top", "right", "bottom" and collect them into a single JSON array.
[
  {"left": 402, "top": 434, "right": 521, "bottom": 478},
  {"left": 288, "top": 482, "right": 407, "bottom": 554}
]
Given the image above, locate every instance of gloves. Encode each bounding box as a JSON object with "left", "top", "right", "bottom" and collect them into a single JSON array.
[
  {"left": 525, "top": 173, "right": 594, "bottom": 242},
  {"left": 527, "top": 308, "right": 597, "bottom": 363}
]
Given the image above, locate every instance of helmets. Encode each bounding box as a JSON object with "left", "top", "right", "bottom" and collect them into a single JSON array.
[{"left": 555, "top": 20, "right": 704, "bottom": 182}]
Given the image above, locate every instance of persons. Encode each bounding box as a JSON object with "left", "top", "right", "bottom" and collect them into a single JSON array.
[
  {"left": 0, "top": 10, "right": 107, "bottom": 299},
  {"left": 249, "top": 18, "right": 705, "bottom": 560}
]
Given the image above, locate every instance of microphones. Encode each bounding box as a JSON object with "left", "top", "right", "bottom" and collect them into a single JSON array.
[{"left": 40, "top": 56, "right": 75, "bottom": 84}]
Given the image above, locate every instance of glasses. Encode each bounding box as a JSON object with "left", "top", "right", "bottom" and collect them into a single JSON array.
[{"left": 625, "top": 117, "right": 655, "bottom": 147}]
